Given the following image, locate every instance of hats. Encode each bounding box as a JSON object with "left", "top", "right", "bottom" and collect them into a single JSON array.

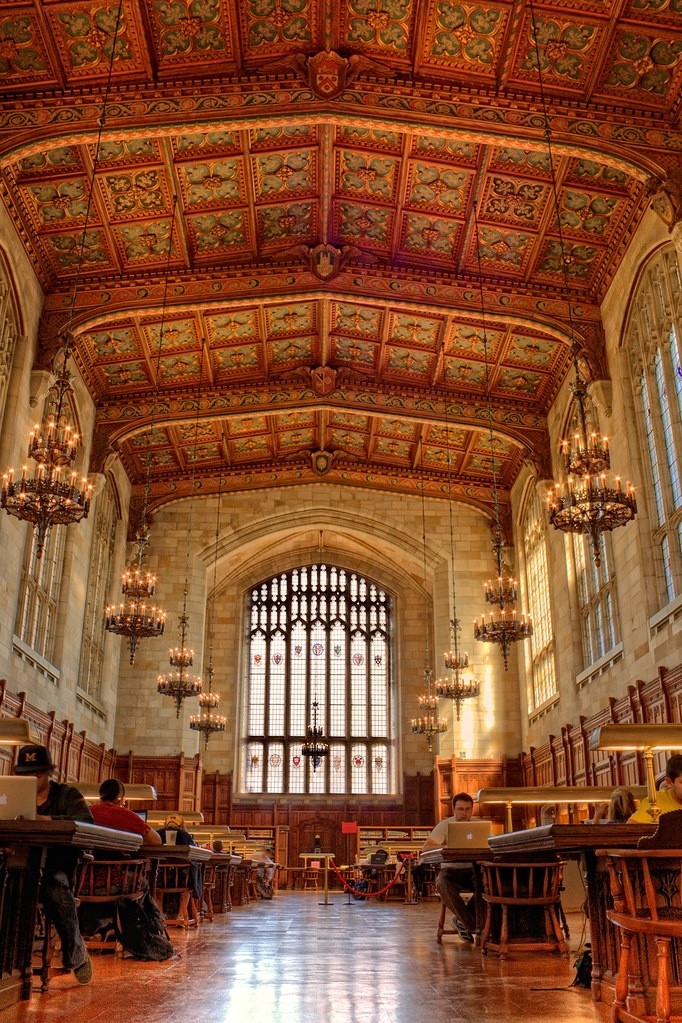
[
  {"left": 13, "top": 744, "right": 52, "bottom": 772},
  {"left": 166, "top": 813, "right": 182, "bottom": 828}
]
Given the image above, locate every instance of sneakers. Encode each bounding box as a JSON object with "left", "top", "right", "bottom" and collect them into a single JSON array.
[{"left": 450, "top": 916, "right": 474, "bottom": 944}]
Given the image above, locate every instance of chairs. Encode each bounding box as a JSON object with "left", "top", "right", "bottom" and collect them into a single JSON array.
[
  {"left": 429, "top": 860, "right": 570, "bottom": 960},
  {"left": 595, "top": 848, "right": 682, "bottom": 1023},
  {"left": 32, "top": 851, "right": 198, "bottom": 990},
  {"left": 384, "top": 869, "right": 412, "bottom": 902},
  {"left": 304, "top": 871, "right": 319, "bottom": 891}
]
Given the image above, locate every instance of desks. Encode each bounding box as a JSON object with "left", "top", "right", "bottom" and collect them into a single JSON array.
[
  {"left": 420, "top": 822, "right": 682, "bottom": 1023},
  {"left": 140, "top": 819, "right": 283, "bottom": 913},
  {"left": 0, "top": 819, "right": 142, "bottom": 1011}
]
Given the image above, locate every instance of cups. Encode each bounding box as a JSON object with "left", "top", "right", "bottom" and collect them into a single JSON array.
[
  {"left": 585, "top": 819, "right": 609, "bottom": 824},
  {"left": 165, "top": 830, "right": 177, "bottom": 845}
]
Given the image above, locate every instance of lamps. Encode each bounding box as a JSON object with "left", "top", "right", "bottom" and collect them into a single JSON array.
[
  {"left": 531, "top": 1, "right": 638, "bottom": 567},
  {"left": 190, "top": 433, "right": 225, "bottom": 751},
  {"left": 435, "top": 343, "right": 478, "bottom": 720},
  {"left": 0, "top": 774, "right": 265, "bottom": 860},
  {"left": 106, "top": 194, "right": 179, "bottom": 665},
  {"left": 361, "top": 840, "right": 428, "bottom": 862},
  {"left": 472, "top": 200, "right": 534, "bottom": 673},
  {"left": 299, "top": 853, "right": 335, "bottom": 868},
  {"left": 302, "top": 702, "right": 328, "bottom": 773},
  {"left": 1, "top": 0, "right": 122, "bottom": 560},
  {"left": 406, "top": 436, "right": 447, "bottom": 750},
  {"left": 475, "top": 786, "right": 648, "bottom": 834},
  {"left": 157, "top": 338, "right": 206, "bottom": 720},
  {"left": 0, "top": 717, "right": 39, "bottom": 746},
  {"left": 588, "top": 723, "right": 682, "bottom": 824}
]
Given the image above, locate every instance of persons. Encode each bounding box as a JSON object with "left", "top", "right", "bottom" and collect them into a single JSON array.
[
  {"left": 211, "top": 841, "right": 228, "bottom": 854},
  {"left": 625, "top": 753, "right": 682, "bottom": 824},
  {"left": 73, "top": 779, "right": 149, "bottom": 954},
  {"left": 584, "top": 788, "right": 638, "bottom": 920},
  {"left": 421, "top": 793, "right": 500, "bottom": 943},
  {"left": 226, "top": 846, "right": 244, "bottom": 859},
  {"left": 150, "top": 814, "right": 202, "bottom": 928},
  {"left": 1, "top": 744, "right": 92, "bottom": 983}
]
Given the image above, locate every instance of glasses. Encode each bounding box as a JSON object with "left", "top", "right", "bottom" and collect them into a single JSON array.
[{"left": 455, "top": 807, "right": 473, "bottom": 814}]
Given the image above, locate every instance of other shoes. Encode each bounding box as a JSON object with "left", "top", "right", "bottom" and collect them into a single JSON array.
[
  {"left": 73, "top": 953, "right": 93, "bottom": 983},
  {"left": 83, "top": 923, "right": 115, "bottom": 955}
]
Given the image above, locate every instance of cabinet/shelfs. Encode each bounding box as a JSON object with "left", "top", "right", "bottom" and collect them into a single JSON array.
[{"left": 357, "top": 825, "right": 433, "bottom": 862}]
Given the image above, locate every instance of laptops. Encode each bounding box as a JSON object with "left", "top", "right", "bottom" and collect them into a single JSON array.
[
  {"left": 130, "top": 810, "right": 146, "bottom": 821},
  {"left": 0, "top": 775, "right": 38, "bottom": 820},
  {"left": 445, "top": 820, "right": 492, "bottom": 847}
]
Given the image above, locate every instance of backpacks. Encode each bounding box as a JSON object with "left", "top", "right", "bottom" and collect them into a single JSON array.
[{"left": 113, "top": 894, "right": 176, "bottom": 961}]
